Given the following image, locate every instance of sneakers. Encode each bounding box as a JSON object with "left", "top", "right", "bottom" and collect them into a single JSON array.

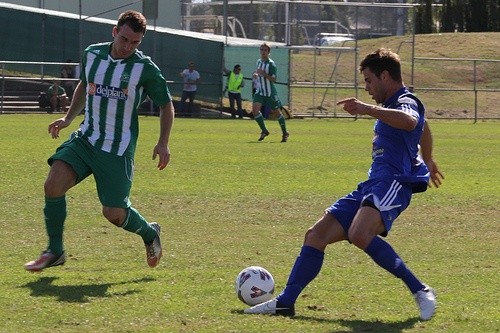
[
  {"left": 23, "top": 247, "right": 67, "bottom": 271},
  {"left": 241, "top": 297, "right": 296, "bottom": 317},
  {"left": 144, "top": 222, "right": 162, "bottom": 268},
  {"left": 281, "top": 132, "right": 290, "bottom": 142},
  {"left": 412, "top": 282, "right": 437, "bottom": 320},
  {"left": 258, "top": 130, "right": 269, "bottom": 141}
]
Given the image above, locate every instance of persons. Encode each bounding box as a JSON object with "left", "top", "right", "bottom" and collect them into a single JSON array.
[
  {"left": 223, "top": 56, "right": 244, "bottom": 118},
  {"left": 47, "top": 59, "right": 81, "bottom": 113},
  {"left": 23, "top": 10, "right": 174, "bottom": 272},
  {"left": 178, "top": 62, "right": 200, "bottom": 117},
  {"left": 244, "top": 48, "right": 445, "bottom": 321},
  {"left": 252, "top": 44, "right": 290, "bottom": 142}
]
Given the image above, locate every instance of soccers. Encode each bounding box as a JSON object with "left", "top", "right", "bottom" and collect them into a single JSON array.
[{"left": 234, "top": 265, "right": 275, "bottom": 307}]
